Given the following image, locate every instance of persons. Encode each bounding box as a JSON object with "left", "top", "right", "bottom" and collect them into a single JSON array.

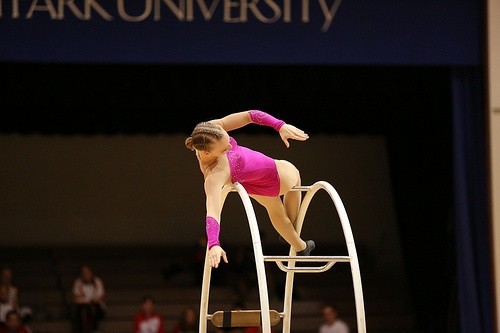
[
  {"left": 185, "top": 110, "right": 316, "bottom": 268},
  {"left": 0, "top": 265, "right": 351, "bottom": 333}
]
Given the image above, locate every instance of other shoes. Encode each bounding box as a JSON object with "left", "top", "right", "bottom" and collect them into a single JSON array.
[
  {"left": 279, "top": 235, "right": 287, "bottom": 244},
  {"left": 297, "top": 240, "right": 315, "bottom": 256}
]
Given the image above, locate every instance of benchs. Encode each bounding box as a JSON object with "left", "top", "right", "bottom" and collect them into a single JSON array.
[{"left": 0, "top": 259, "right": 355, "bottom": 333}]
